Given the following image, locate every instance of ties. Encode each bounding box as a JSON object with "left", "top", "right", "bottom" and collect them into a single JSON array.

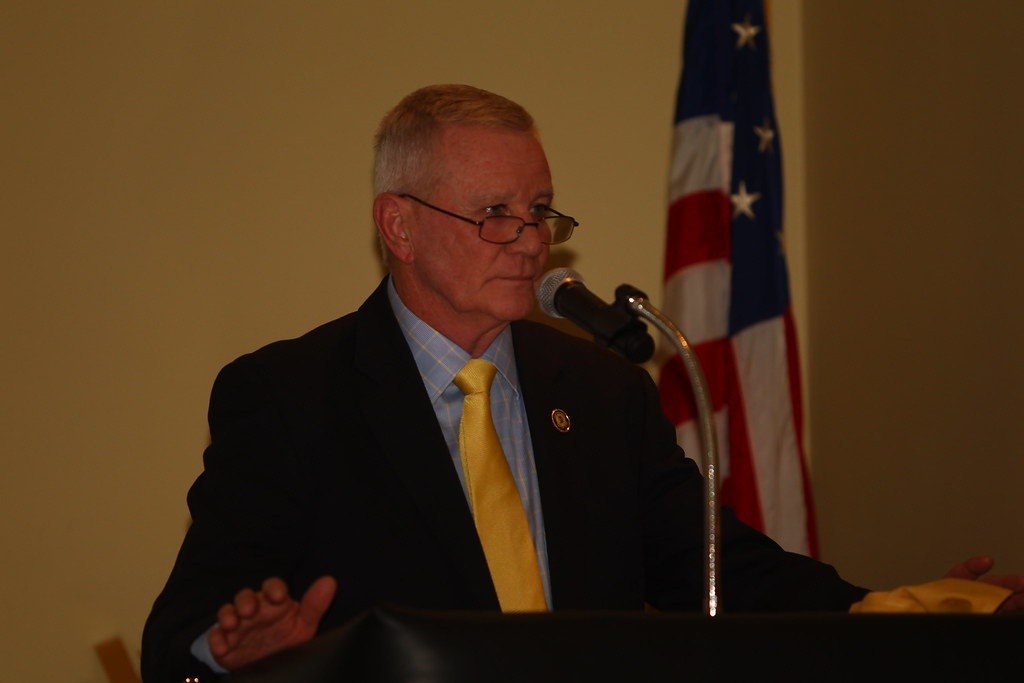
[{"left": 452, "top": 359, "right": 548, "bottom": 615}]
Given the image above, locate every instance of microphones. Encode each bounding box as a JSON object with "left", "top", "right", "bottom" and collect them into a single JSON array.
[{"left": 537, "top": 267, "right": 655, "bottom": 364}]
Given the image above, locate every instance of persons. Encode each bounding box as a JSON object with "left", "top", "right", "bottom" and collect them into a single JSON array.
[{"left": 138, "top": 84, "right": 1024, "bottom": 683}]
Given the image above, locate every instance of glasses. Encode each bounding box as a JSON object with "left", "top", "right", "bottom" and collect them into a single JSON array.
[{"left": 395, "top": 193, "right": 579, "bottom": 245}]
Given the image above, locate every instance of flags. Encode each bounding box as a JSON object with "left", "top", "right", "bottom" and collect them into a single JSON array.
[{"left": 660, "top": 0, "right": 819, "bottom": 562}]
